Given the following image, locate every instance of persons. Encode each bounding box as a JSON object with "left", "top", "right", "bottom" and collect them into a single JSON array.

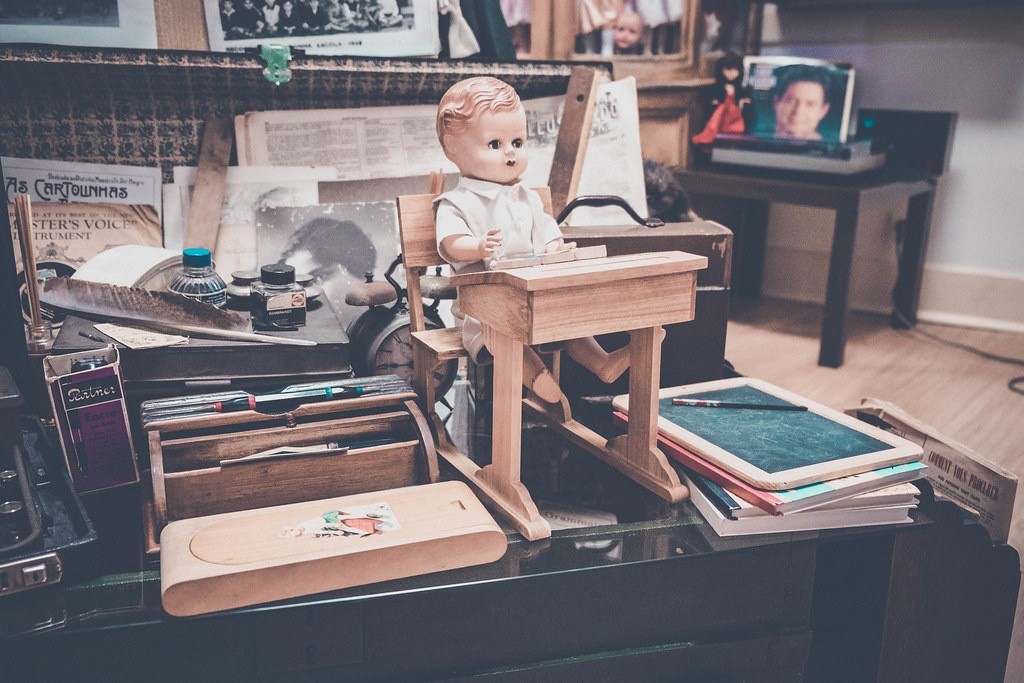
[
  {"left": 770, "top": 66, "right": 837, "bottom": 140},
  {"left": 281, "top": 219, "right": 378, "bottom": 330},
  {"left": 437, "top": 76, "right": 631, "bottom": 404},
  {"left": 219, "top": 0, "right": 381, "bottom": 41}
]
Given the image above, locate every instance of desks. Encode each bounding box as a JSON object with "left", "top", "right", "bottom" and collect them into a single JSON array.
[
  {"left": 670, "top": 163, "right": 946, "bottom": 368},
  {"left": 451, "top": 252, "right": 708, "bottom": 542}
]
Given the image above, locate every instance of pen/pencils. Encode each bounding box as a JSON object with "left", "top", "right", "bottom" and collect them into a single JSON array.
[
  {"left": 672, "top": 397, "right": 809, "bottom": 410},
  {"left": 208, "top": 385, "right": 380, "bottom": 412}
]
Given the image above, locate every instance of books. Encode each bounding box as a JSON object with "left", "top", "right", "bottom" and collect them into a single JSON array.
[{"left": 612, "top": 413, "right": 928, "bottom": 538}]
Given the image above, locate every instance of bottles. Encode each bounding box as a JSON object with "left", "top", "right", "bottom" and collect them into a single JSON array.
[
  {"left": 166, "top": 247, "right": 227, "bottom": 312},
  {"left": 250, "top": 262, "right": 307, "bottom": 331}
]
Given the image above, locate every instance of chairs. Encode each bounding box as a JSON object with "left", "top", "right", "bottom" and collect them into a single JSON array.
[{"left": 394, "top": 186, "right": 572, "bottom": 445}]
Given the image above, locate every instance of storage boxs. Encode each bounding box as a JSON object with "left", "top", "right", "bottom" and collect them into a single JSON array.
[{"left": 43, "top": 344, "right": 138, "bottom": 495}]
